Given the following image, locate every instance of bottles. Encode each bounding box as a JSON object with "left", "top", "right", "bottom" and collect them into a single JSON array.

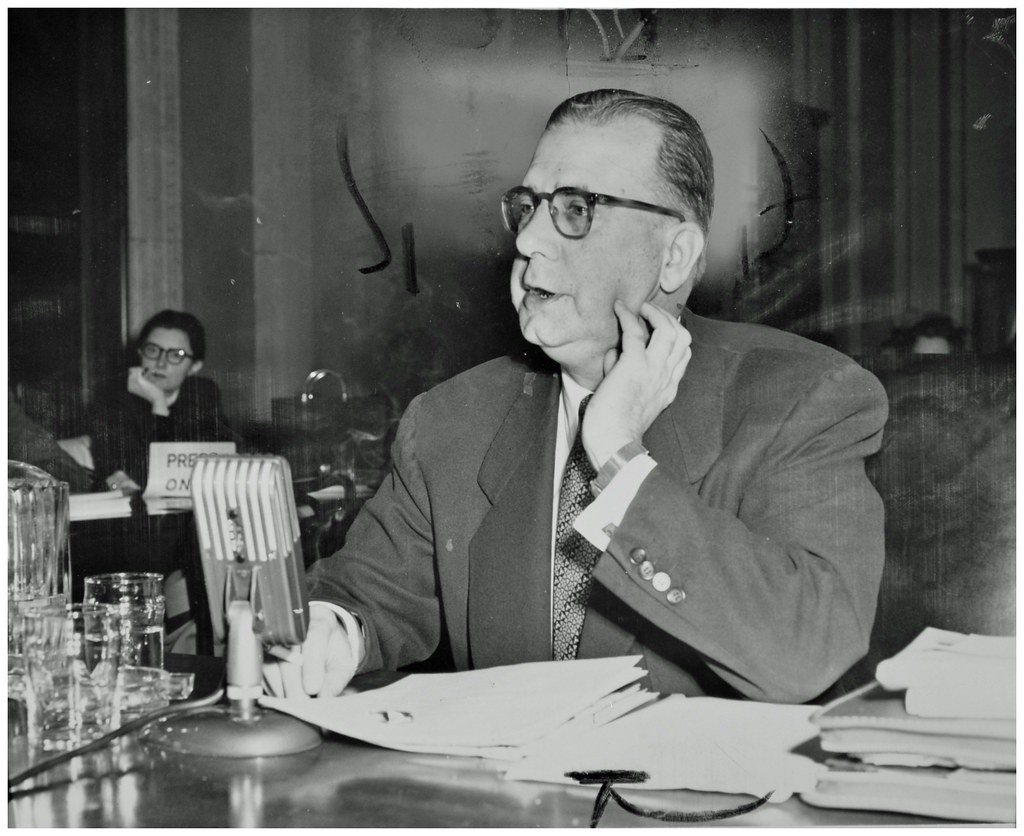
[{"left": 7, "top": 456, "right": 193, "bottom": 768}]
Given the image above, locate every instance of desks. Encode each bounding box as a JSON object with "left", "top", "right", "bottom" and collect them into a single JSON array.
[{"left": 9, "top": 700, "right": 1016, "bottom": 825}]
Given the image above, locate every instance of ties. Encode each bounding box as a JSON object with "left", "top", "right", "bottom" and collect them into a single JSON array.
[{"left": 551, "top": 394, "right": 600, "bottom": 658}]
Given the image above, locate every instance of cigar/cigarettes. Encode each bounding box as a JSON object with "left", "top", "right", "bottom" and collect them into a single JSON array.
[{"left": 261, "top": 640, "right": 303, "bottom": 666}]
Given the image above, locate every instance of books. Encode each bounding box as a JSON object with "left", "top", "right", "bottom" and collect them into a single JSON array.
[
  {"left": 68, "top": 489, "right": 131, "bottom": 521},
  {"left": 257, "top": 624, "right": 1016, "bottom": 827}
]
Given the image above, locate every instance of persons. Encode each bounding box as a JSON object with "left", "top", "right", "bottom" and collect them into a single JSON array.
[
  {"left": 9, "top": 384, "right": 94, "bottom": 490},
  {"left": 253, "top": 89, "right": 889, "bottom": 702},
  {"left": 89, "top": 307, "right": 224, "bottom": 578}
]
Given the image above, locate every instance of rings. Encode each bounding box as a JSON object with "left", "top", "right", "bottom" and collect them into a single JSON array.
[{"left": 111, "top": 483, "right": 117, "bottom": 490}]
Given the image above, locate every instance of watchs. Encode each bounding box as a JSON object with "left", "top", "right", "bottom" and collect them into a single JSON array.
[{"left": 588, "top": 440, "right": 650, "bottom": 499}]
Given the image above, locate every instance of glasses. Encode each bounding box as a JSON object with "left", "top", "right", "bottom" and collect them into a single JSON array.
[
  {"left": 138, "top": 340, "right": 197, "bottom": 366},
  {"left": 499, "top": 185, "right": 686, "bottom": 238}
]
